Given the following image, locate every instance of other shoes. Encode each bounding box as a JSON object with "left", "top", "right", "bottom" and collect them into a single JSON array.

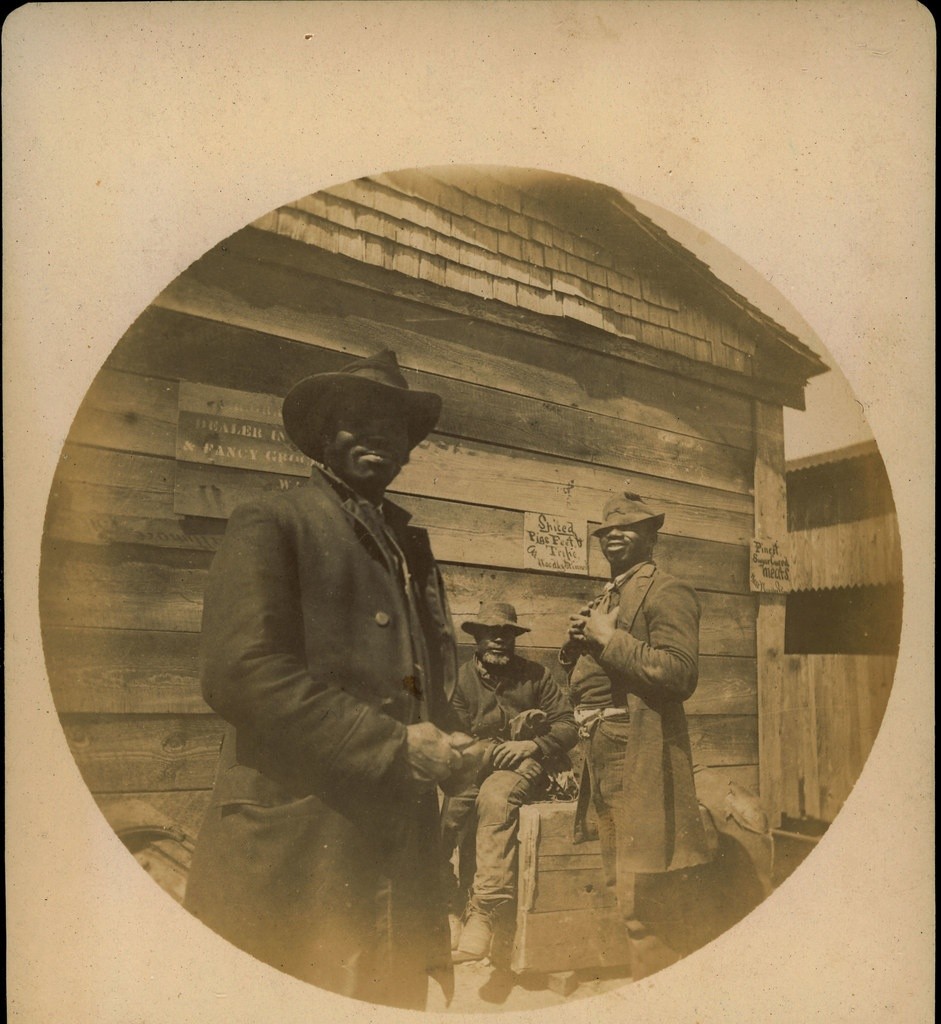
[
  {"left": 459, "top": 898, "right": 495, "bottom": 956},
  {"left": 446, "top": 894, "right": 465, "bottom": 950}
]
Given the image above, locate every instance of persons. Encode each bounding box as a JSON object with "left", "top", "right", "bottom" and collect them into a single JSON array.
[
  {"left": 182, "top": 347, "right": 479, "bottom": 1012},
  {"left": 557, "top": 491, "right": 713, "bottom": 984},
  {"left": 440, "top": 601, "right": 578, "bottom": 960}
]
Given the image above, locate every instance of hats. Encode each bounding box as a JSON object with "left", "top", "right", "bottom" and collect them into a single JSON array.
[
  {"left": 461, "top": 602, "right": 532, "bottom": 637},
  {"left": 283, "top": 343, "right": 441, "bottom": 467},
  {"left": 591, "top": 492, "right": 665, "bottom": 537}
]
